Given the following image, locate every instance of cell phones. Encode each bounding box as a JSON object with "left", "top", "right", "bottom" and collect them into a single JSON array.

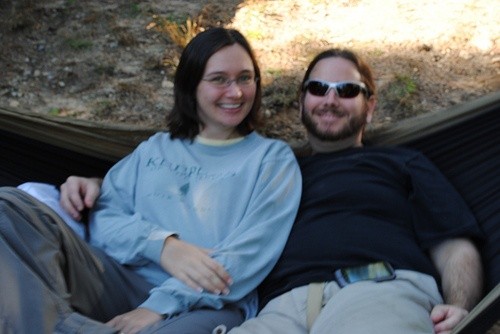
[{"left": 333, "top": 260, "right": 396, "bottom": 287}]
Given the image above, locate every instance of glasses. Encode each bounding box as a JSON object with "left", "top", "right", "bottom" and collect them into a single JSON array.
[
  {"left": 303, "top": 80, "right": 368, "bottom": 99},
  {"left": 201, "top": 72, "right": 260, "bottom": 89}
]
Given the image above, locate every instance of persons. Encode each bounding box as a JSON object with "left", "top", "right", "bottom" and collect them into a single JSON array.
[
  {"left": 0, "top": 28, "right": 303, "bottom": 334},
  {"left": 60, "top": 50, "right": 483, "bottom": 334}
]
